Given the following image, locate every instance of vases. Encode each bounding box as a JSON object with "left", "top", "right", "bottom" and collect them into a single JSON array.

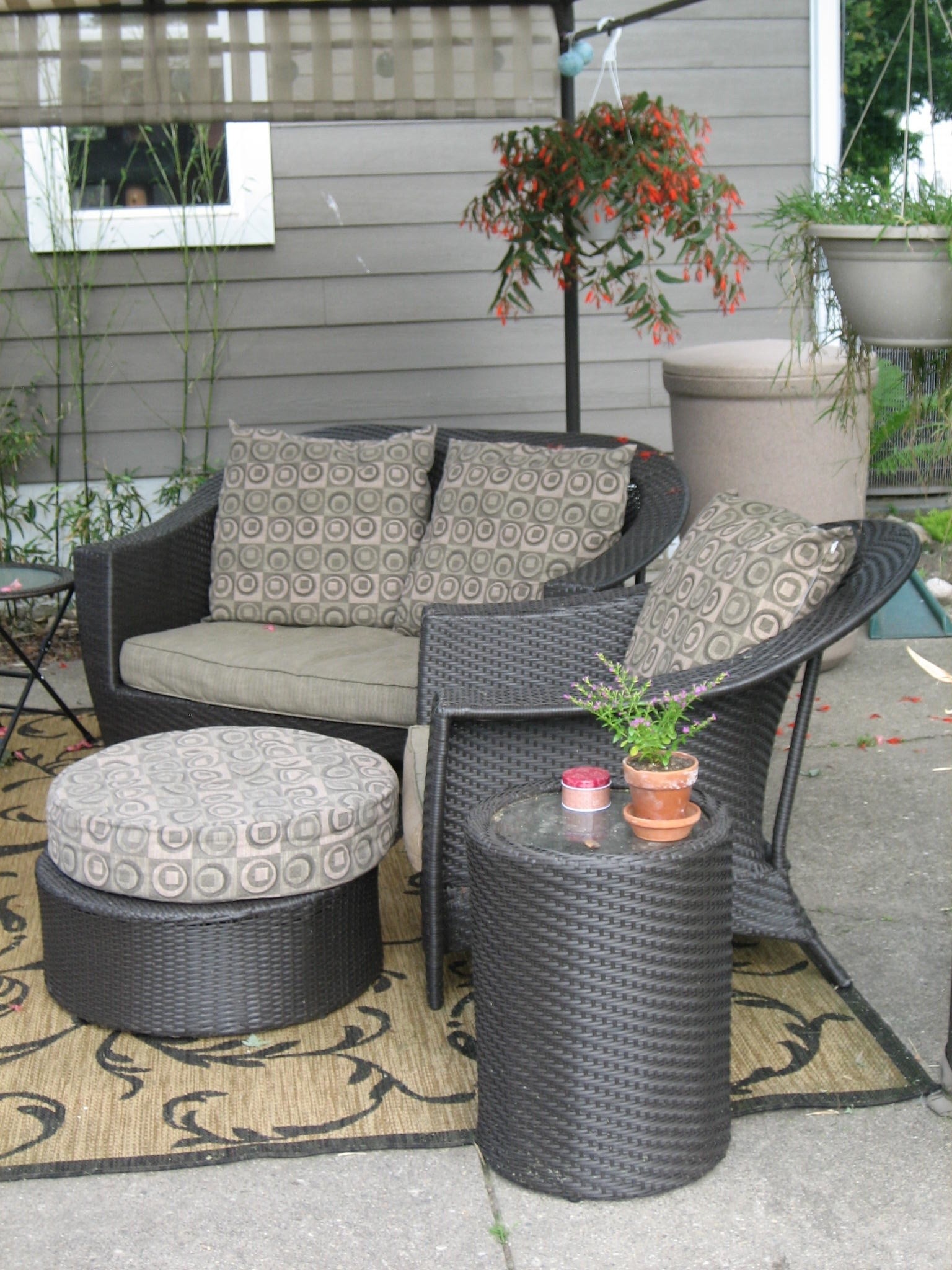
[
  {"left": 622, "top": 749, "right": 699, "bottom": 820},
  {"left": 572, "top": 177, "right": 624, "bottom": 242}
]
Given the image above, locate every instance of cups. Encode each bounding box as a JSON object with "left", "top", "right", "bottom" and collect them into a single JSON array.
[{"left": 124, "top": 184, "right": 148, "bottom": 206}]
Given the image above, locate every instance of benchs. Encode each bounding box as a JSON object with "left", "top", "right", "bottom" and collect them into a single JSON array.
[{"left": 71, "top": 423, "right": 689, "bottom": 763}]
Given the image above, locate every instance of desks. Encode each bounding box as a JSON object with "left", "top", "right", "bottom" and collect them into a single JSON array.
[
  {"left": 0, "top": 560, "right": 95, "bottom": 761},
  {"left": 463, "top": 775, "right": 733, "bottom": 1202}
]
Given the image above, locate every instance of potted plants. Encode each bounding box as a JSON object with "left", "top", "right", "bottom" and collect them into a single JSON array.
[{"left": 753, "top": 162, "right": 952, "bottom": 494}]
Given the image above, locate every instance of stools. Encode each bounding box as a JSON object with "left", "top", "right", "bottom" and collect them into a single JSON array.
[{"left": 33, "top": 725, "right": 401, "bottom": 1040}]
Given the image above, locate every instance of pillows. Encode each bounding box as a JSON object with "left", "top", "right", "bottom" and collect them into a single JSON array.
[
  {"left": 201, "top": 422, "right": 438, "bottom": 629},
  {"left": 623, "top": 488, "right": 859, "bottom": 683},
  {"left": 393, "top": 436, "right": 639, "bottom": 637}
]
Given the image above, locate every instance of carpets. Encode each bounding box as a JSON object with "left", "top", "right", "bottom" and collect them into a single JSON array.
[{"left": 0, "top": 707, "right": 942, "bottom": 1179}]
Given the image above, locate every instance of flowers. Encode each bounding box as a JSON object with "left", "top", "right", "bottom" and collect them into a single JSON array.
[
  {"left": 461, "top": 87, "right": 752, "bottom": 347},
  {"left": 563, "top": 652, "right": 731, "bottom": 767}
]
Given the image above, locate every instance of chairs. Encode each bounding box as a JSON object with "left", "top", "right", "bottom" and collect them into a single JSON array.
[{"left": 402, "top": 518, "right": 923, "bottom": 1011}]
